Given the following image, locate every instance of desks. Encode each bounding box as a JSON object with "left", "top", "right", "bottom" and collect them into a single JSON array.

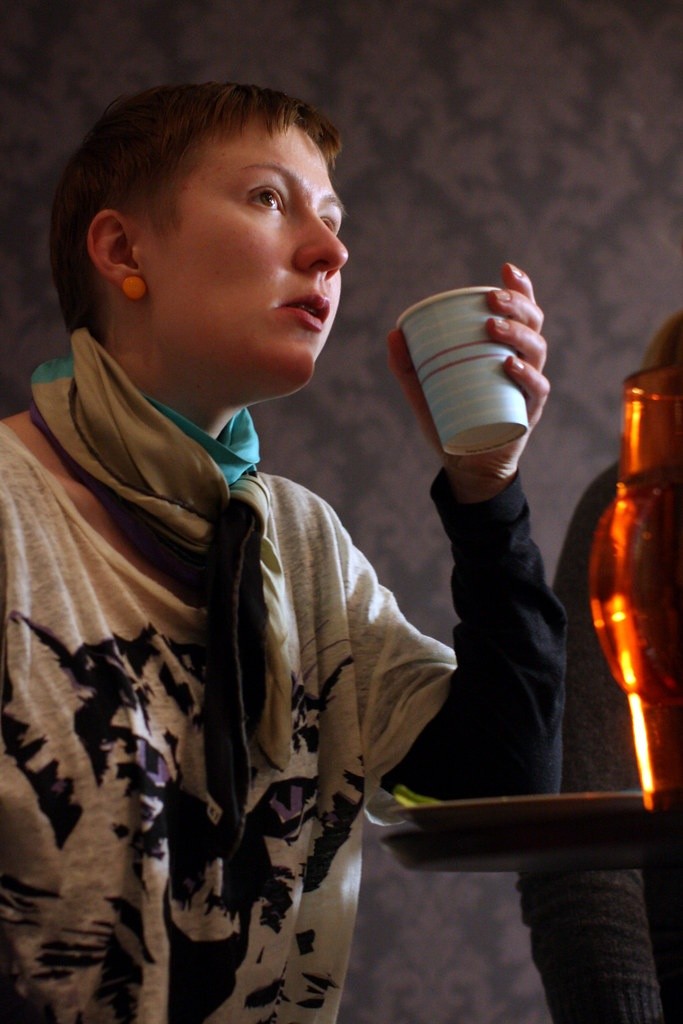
[{"left": 360, "top": 783, "right": 683, "bottom": 1024}]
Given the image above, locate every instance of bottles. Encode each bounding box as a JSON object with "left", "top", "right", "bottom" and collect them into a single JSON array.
[{"left": 588, "top": 363, "right": 683, "bottom": 810}]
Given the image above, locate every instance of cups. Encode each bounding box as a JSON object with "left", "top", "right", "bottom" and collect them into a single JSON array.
[{"left": 394, "top": 285, "right": 529, "bottom": 457}]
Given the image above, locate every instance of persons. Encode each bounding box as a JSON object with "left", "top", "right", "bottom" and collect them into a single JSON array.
[
  {"left": 0, "top": 81, "right": 571, "bottom": 1024},
  {"left": 516, "top": 309, "right": 682, "bottom": 1023}
]
